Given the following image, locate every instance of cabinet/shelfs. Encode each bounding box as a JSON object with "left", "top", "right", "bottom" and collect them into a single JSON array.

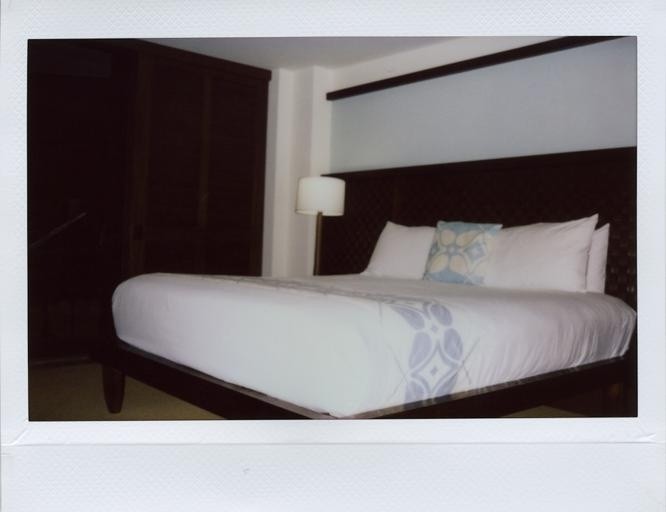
[
  {"left": 29, "top": 40, "right": 151, "bottom": 370},
  {"left": 129, "top": 75, "right": 266, "bottom": 281}
]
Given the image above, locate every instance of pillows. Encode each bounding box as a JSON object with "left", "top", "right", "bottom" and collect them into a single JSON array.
[{"left": 360, "top": 214, "right": 610, "bottom": 294}]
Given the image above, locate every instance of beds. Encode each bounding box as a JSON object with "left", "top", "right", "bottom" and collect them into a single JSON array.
[{"left": 99, "top": 146, "right": 637, "bottom": 419}]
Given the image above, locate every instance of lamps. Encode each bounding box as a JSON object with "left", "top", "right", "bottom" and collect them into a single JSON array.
[{"left": 295, "top": 177, "right": 346, "bottom": 275}]
